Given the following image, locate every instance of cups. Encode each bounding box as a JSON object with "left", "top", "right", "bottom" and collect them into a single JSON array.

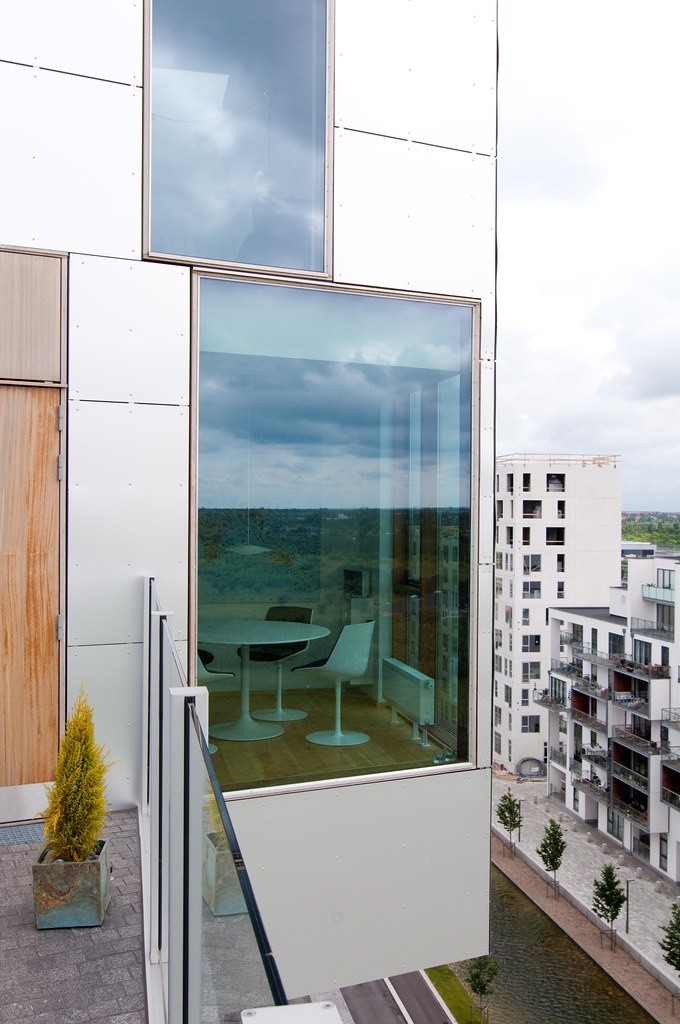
[
  {"left": 444, "top": 748, "right": 457, "bottom": 763},
  {"left": 433, "top": 749, "right": 444, "bottom": 764}
]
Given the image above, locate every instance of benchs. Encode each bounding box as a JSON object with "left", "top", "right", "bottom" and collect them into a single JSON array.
[{"left": 615, "top": 690, "right": 636, "bottom": 702}]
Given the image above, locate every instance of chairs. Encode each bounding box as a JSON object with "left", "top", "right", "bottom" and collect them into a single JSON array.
[
  {"left": 289, "top": 619, "right": 376, "bottom": 747},
  {"left": 197, "top": 648, "right": 236, "bottom": 755},
  {"left": 236, "top": 604, "right": 314, "bottom": 724}
]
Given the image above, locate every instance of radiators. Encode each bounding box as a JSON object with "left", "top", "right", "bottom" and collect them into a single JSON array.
[{"left": 380, "top": 657, "right": 438, "bottom": 748}]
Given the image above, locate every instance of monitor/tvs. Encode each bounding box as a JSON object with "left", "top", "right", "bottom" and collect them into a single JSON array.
[{"left": 340, "top": 566, "right": 380, "bottom": 598}]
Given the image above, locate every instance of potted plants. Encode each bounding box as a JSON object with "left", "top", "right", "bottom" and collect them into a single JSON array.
[
  {"left": 202, "top": 782, "right": 250, "bottom": 917},
  {"left": 29, "top": 682, "right": 116, "bottom": 933}
]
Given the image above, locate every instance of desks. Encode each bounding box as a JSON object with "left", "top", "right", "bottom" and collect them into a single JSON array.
[{"left": 198, "top": 618, "right": 332, "bottom": 742}]
